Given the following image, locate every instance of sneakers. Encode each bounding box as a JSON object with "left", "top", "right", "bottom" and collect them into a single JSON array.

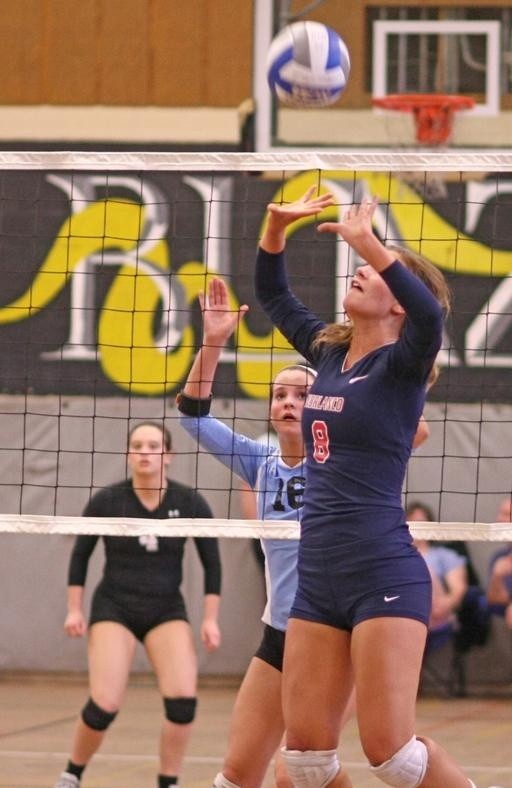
[{"left": 55, "top": 771, "right": 80, "bottom": 788}]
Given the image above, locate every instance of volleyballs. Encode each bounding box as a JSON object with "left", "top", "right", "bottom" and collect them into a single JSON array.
[{"left": 266, "top": 20, "right": 352, "bottom": 108}]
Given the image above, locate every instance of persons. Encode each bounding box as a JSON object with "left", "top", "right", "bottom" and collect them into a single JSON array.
[
  {"left": 256, "top": 181, "right": 479, "bottom": 788},
  {"left": 174, "top": 274, "right": 429, "bottom": 786},
  {"left": 48, "top": 416, "right": 227, "bottom": 787},
  {"left": 402, "top": 500, "right": 468, "bottom": 638},
  {"left": 481, "top": 498, "right": 511, "bottom": 631}
]
